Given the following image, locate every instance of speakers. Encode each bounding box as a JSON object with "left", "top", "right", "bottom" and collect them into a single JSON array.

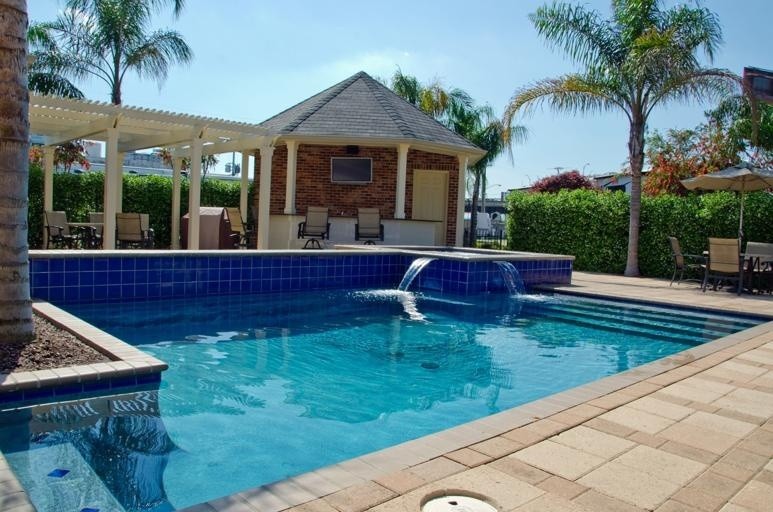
[{"left": 347, "top": 145, "right": 359, "bottom": 154}]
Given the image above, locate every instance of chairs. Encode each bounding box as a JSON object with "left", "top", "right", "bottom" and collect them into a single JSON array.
[
  {"left": 297, "top": 204, "right": 331, "bottom": 252},
  {"left": 225, "top": 206, "right": 258, "bottom": 250},
  {"left": 354, "top": 206, "right": 387, "bottom": 249},
  {"left": 663, "top": 231, "right": 773, "bottom": 297},
  {"left": 40, "top": 210, "right": 161, "bottom": 248}
]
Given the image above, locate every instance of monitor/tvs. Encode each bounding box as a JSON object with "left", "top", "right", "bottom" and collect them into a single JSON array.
[{"left": 330, "top": 157, "right": 373, "bottom": 185}]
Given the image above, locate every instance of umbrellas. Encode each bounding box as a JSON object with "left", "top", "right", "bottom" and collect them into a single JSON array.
[{"left": 678, "top": 162, "right": 773, "bottom": 253}]
